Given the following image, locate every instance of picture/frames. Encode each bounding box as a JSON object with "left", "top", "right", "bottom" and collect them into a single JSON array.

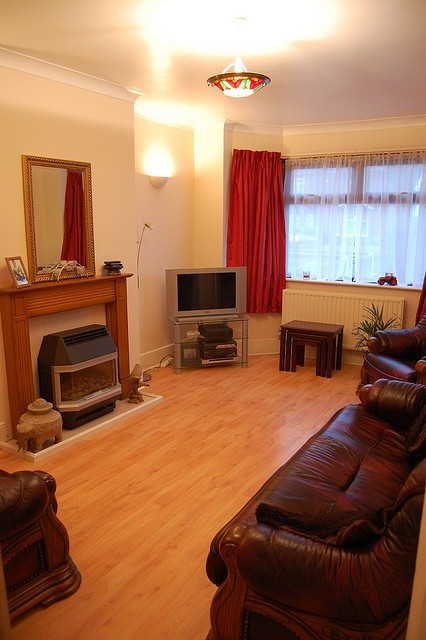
[{"left": 5, "top": 255, "right": 30, "bottom": 289}]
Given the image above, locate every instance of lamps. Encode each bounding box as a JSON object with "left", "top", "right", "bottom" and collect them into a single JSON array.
[
  {"left": 142, "top": 144, "right": 175, "bottom": 189},
  {"left": 206, "top": 17, "right": 271, "bottom": 98}
]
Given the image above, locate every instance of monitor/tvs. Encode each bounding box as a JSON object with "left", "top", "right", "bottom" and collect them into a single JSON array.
[{"left": 165, "top": 268, "right": 247, "bottom": 318}]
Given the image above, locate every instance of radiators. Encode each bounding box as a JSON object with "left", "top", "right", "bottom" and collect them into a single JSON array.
[{"left": 282, "top": 288, "right": 406, "bottom": 354}]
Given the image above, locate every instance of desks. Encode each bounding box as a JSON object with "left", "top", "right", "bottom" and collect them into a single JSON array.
[{"left": 165, "top": 313, "right": 249, "bottom": 376}]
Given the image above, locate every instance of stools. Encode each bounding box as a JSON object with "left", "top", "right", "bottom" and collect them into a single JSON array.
[{"left": 279, "top": 320, "right": 344, "bottom": 378}]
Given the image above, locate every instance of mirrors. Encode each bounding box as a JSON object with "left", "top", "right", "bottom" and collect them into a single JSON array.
[{"left": 21, "top": 154, "right": 96, "bottom": 284}]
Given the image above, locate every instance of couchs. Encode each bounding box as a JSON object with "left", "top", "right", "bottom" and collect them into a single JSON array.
[
  {"left": 0, "top": 470, "right": 82, "bottom": 640},
  {"left": 206, "top": 378, "right": 425, "bottom": 640},
  {"left": 355, "top": 311, "right": 426, "bottom": 396}
]
